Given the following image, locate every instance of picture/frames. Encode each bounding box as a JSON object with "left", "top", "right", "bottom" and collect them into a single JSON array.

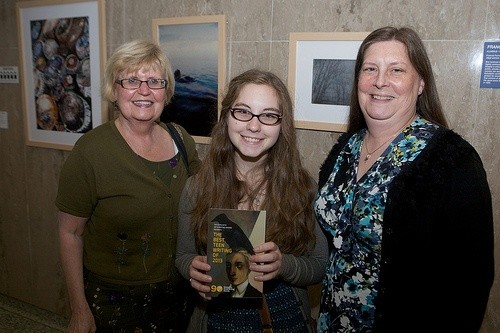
[
  {"left": 152, "top": 14, "right": 226, "bottom": 145},
  {"left": 288, "top": 31, "right": 373, "bottom": 133},
  {"left": 16, "top": 0, "right": 106, "bottom": 152}
]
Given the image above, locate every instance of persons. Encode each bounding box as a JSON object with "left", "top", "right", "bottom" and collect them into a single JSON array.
[
  {"left": 54, "top": 40, "right": 201, "bottom": 333},
  {"left": 312, "top": 26, "right": 495, "bottom": 333},
  {"left": 175, "top": 69, "right": 329, "bottom": 333}
]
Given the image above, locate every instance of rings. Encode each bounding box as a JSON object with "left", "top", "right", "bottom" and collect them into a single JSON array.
[{"left": 189, "top": 278, "right": 195, "bottom": 283}]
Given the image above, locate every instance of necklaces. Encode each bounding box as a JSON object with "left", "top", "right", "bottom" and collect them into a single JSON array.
[{"left": 364, "top": 112, "right": 415, "bottom": 162}]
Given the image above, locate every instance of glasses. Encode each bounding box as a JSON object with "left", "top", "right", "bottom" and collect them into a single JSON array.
[
  {"left": 115, "top": 78, "right": 168, "bottom": 90},
  {"left": 229, "top": 107, "right": 282, "bottom": 125}
]
